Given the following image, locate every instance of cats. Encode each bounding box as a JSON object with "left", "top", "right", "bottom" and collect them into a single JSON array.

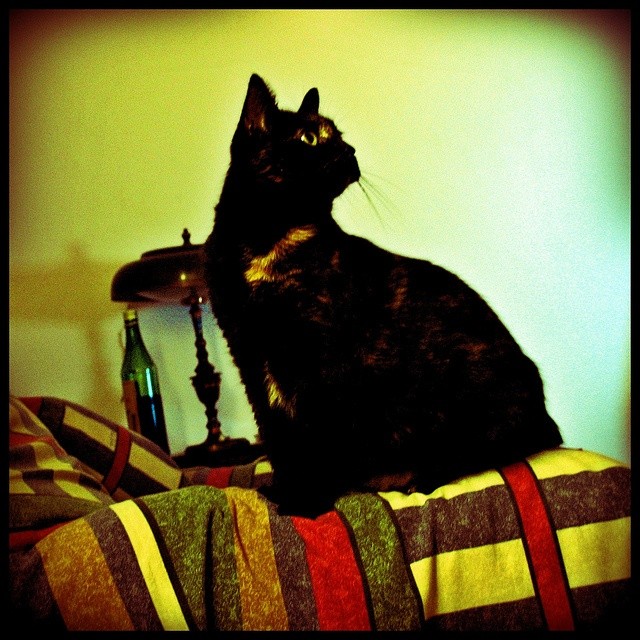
[{"left": 200, "top": 71, "right": 563, "bottom": 520}]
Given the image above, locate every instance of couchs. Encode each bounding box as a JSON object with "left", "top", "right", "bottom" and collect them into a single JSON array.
[{"left": 8, "top": 392, "right": 630, "bottom": 633}]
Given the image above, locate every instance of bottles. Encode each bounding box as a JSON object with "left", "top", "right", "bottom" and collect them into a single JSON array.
[{"left": 120, "top": 307, "right": 170, "bottom": 454}]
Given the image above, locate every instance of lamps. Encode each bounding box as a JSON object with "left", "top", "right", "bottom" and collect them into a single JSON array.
[{"left": 110, "top": 227, "right": 252, "bottom": 465}]
